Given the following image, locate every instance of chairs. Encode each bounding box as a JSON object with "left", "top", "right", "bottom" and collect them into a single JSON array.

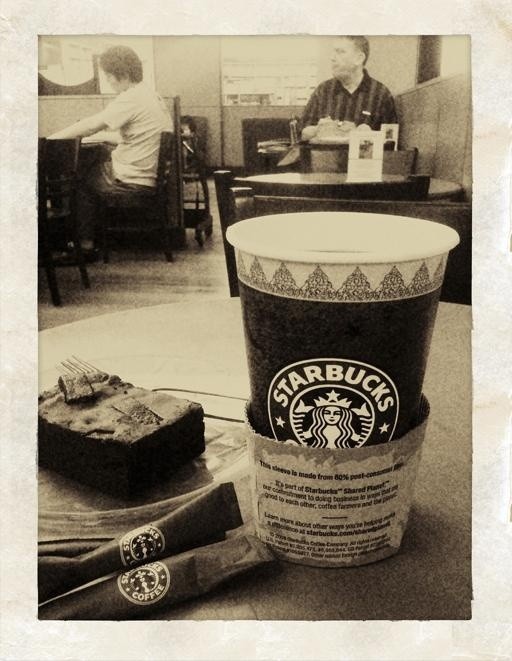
[
  {"left": 97, "top": 131, "right": 176, "bottom": 265},
  {"left": 179, "top": 114, "right": 213, "bottom": 249},
  {"left": 240, "top": 117, "right": 297, "bottom": 177},
  {"left": 295, "top": 139, "right": 394, "bottom": 174},
  {"left": 228, "top": 185, "right": 469, "bottom": 307},
  {"left": 39, "top": 135, "right": 92, "bottom": 304},
  {"left": 215, "top": 168, "right": 431, "bottom": 297},
  {"left": 301, "top": 142, "right": 418, "bottom": 175}
]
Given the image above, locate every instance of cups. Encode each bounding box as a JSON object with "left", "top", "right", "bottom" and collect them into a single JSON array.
[
  {"left": 224, "top": 210, "right": 462, "bottom": 567},
  {"left": 288, "top": 111, "right": 304, "bottom": 142}
]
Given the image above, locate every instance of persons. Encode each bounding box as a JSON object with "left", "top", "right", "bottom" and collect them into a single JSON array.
[
  {"left": 45, "top": 44, "right": 173, "bottom": 267},
  {"left": 296, "top": 35, "right": 397, "bottom": 133},
  {"left": 180, "top": 114, "right": 197, "bottom": 170}
]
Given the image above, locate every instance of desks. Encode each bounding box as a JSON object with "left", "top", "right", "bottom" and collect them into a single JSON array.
[{"left": 232, "top": 171, "right": 463, "bottom": 201}]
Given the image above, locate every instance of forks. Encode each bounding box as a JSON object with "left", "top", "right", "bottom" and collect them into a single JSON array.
[{"left": 54, "top": 355, "right": 251, "bottom": 428}]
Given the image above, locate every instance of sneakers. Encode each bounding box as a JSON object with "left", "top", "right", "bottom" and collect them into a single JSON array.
[{"left": 67, "top": 241, "right": 97, "bottom": 262}]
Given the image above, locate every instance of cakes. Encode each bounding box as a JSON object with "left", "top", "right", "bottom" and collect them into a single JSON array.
[{"left": 39, "top": 371, "right": 205, "bottom": 496}]
